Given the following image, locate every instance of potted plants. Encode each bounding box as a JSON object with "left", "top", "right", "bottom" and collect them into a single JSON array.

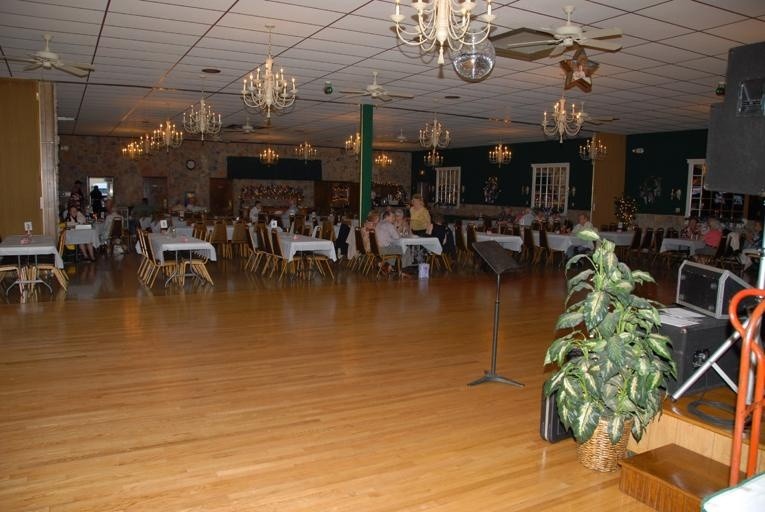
[{"left": 542, "top": 227, "right": 677, "bottom": 473}]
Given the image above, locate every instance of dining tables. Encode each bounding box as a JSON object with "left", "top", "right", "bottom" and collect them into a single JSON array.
[{"left": 542, "top": 227, "right": 677, "bottom": 473}]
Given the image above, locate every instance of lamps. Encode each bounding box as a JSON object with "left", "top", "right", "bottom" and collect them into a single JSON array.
[
  {"left": 296, "top": 112, "right": 607, "bottom": 176},
  {"left": 540, "top": 85, "right": 584, "bottom": 142},
  {"left": 120, "top": 23, "right": 296, "bottom": 161},
  {"left": 391, "top": 0, "right": 497, "bottom": 65}
]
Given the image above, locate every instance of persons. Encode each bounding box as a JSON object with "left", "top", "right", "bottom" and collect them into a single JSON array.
[
  {"left": 249, "top": 201, "right": 264, "bottom": 222},
  {"left": 60, "top": 181, "right": 155, "bottom": 263},
  {"left": 494, "top": 205, "right": 595, "bottom": 271},
  {"left": 677, "top": 215, "right": 764, "bottom": 273},
  {"left": 365, "top": 190, "right": 449, "bottom": 280}
]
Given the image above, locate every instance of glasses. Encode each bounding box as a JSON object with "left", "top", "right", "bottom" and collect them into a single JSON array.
[
  {"left": 120, "top": 23, "right": 296, "bottom": 161},
  {"left": 296, "top": 112, "right": 607, "bottom": 176},
  {"left": 540, "top": 85, "right": 584, "bottom": 142},
  {"left": 391, "top": 0, "right": 497, "bottom": 65}
]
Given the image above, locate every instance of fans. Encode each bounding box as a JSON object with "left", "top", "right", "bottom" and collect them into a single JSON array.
[
  {"left": 338, "top": 73, "right": 413, "bottom": 102},
  {"left": 1, "top": 34, "right": 95, "bottom": 77},
  {"left": 573, "top": 101, "right": 617, "bottom": 126},
  {"left": 507, "top": 5, "right": 624, "bottom": 51}
]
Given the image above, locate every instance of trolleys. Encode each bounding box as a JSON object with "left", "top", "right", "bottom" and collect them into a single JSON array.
[
  {"left": 1, "top": 34, "right": 95, "bottom": 77},
  {"left": 507, "top": 5, "right": 624, "bottom": 51},
  {"left": 573, "top": 101, "right": 617, "bottom": 126},
  {"left": 338, "top": 73, "right": 413, "bottom": 102}
]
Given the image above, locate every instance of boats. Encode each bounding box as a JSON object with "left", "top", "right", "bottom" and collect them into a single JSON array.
[
  {"left": 703, "top": 102, "right": 724, "bottom": 191},
  {"left": 637, "top": 307, "right": 751, "bottom": 397},
  {"left": 675, "top": 259, "right": 756, "bottom": 321},
  {"left": 724, "top": 40, "right": 765, "bottom": 199}
]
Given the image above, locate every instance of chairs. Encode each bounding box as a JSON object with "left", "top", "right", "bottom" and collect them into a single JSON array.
[
  {"left": 59, "top": 209, "right": 267, "bottom": 260},
  {"left": 136, "top": 226, "right": 218, "bottom": 287},
  {"left": 321, "top": 206, "right": 743, "bottom": 287},
  {"left": 2, "top": 228, "right": 69, "bottom": 299},
  {"left": 82, "top": 254, "right": 100, "bottom": 264},
  {"left": 376, "top": 261, "right": 411, "bottom": 281},
  {"left": 244, "top": 210, "right": 336, "bottom": 284}
]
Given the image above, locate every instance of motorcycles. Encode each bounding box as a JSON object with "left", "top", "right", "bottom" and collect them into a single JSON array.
[
  {"left": 466, "top": 274, "right": 527, "bottom": 392},
  {"left": 668, "top": 223, "right": 765, "bottom": 412}
]
